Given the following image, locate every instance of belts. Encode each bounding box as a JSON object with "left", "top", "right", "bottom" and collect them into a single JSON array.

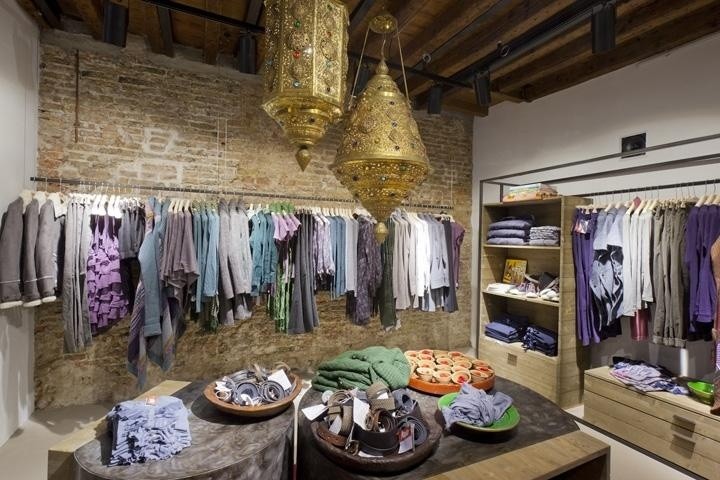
[
  {"left": 226, "top": 369, "right": 285, "bottom": 406},
  {"left": 316, "top": 381, "right": 427, "bottom": 456}
]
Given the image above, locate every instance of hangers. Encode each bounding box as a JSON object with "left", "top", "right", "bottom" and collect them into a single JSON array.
[
  {"left": 574, "top": 178, "right": 720, "bottom": 216},
  {"left": 20, "top": 175, "right": 457, "bottom": 225}
]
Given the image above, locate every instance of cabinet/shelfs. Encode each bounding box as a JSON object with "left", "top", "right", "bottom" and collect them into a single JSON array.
[
  {"left": 477, "top": 195, "right": 593, "bottom": 411},
  {"left": 583, "top": 365, "right": 720, "bottom": 480},
  {"left": 47, "top": 379, "right": 295, "bottom": 480},
  {"left": 297, "top": 373, "right": 610, "bottom": 480}
]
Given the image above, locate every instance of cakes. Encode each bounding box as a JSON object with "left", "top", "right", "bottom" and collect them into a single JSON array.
[{"left": 405, "top": 349, "right": 493, "bottom": 385}]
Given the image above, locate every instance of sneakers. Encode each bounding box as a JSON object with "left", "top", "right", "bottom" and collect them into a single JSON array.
[{"left": 510, "top": 272, "right": 559, "bottom": 302}]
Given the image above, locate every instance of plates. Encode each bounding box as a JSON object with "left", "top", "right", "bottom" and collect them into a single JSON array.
[
  {"left": 438, "top": 391, "right": 522, "bottom": 433},
  {"left": 686, "top": 380, "right": 715, "bottom": 405}
]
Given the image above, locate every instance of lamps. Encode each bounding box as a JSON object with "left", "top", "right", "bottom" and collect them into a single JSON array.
[
  {"left": 330, "top": 7, "right": 434, "bottom": 244},
  {"left": 260, "top": 0, "right": 349, "bottom": 173}
]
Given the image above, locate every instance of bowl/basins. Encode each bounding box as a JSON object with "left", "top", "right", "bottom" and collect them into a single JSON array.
[
  {"left": 401, "top": 352, "right": 496, "bottom": 395},
  {"left": 316, "top": 399, "right": 443, "bottom": 475},
  {"left": 202, "top": 369, "right": 305, "bottom": 418}
]
